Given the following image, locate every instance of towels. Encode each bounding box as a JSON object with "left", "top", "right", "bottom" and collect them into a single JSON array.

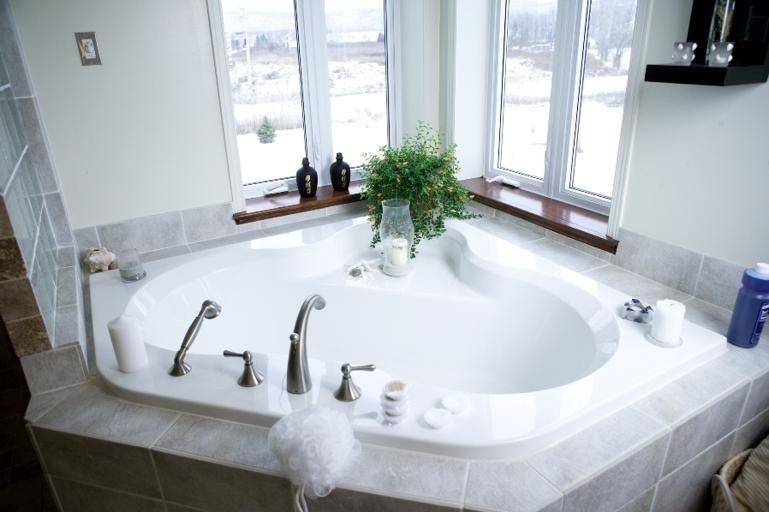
[{"left": 728, "top": 434, "right": 769, "bottom": 511}]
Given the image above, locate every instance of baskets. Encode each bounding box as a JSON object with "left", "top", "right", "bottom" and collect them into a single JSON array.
[{"left": 713, "top": 450, "right": 755, "bottom": 511}]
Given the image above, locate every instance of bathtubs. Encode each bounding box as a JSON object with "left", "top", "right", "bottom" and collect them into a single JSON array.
[{"left": 88, "top": 213, "right": 728, "bottom": 455}]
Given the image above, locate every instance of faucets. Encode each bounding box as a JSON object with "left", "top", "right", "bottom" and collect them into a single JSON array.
[
  {"left": 286, "top": 294, "right": 327, "bottom": 394},
  {"left": 172, "top": 298, "right": 223, "bottom": 378}
]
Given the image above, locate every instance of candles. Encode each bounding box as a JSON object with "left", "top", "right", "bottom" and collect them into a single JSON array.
[
  {"left": 105, "top": 311, "right": 150, "bottom": 375},
  {"left": 650, "top": 297, "right": 688, "bottom": 344},
  {"left": 390, "top": 236, "right": 409, "bottom": 267}
]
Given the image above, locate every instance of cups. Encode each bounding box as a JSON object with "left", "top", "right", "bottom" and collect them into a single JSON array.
[
  {"left": 117, "top": 250, "right": 146, "bottom": 283},
  {"left": 381, "top": 381, "right": 408, "bottom": 425}
]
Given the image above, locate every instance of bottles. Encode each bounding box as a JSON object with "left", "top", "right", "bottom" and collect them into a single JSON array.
[
  {"left": 380, "top": 198, "right": 414, "bottom": 277},
  {"left": 329, "top": 151, "right": 352, "bottom": 190},
  {"left": 295, "top": 158, "right": 318, "bottom": 198}
]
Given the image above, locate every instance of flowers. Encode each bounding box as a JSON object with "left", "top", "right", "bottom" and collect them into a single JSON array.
[{"left": 358, "top": 125, "right": 482, "bottom": 263}]
